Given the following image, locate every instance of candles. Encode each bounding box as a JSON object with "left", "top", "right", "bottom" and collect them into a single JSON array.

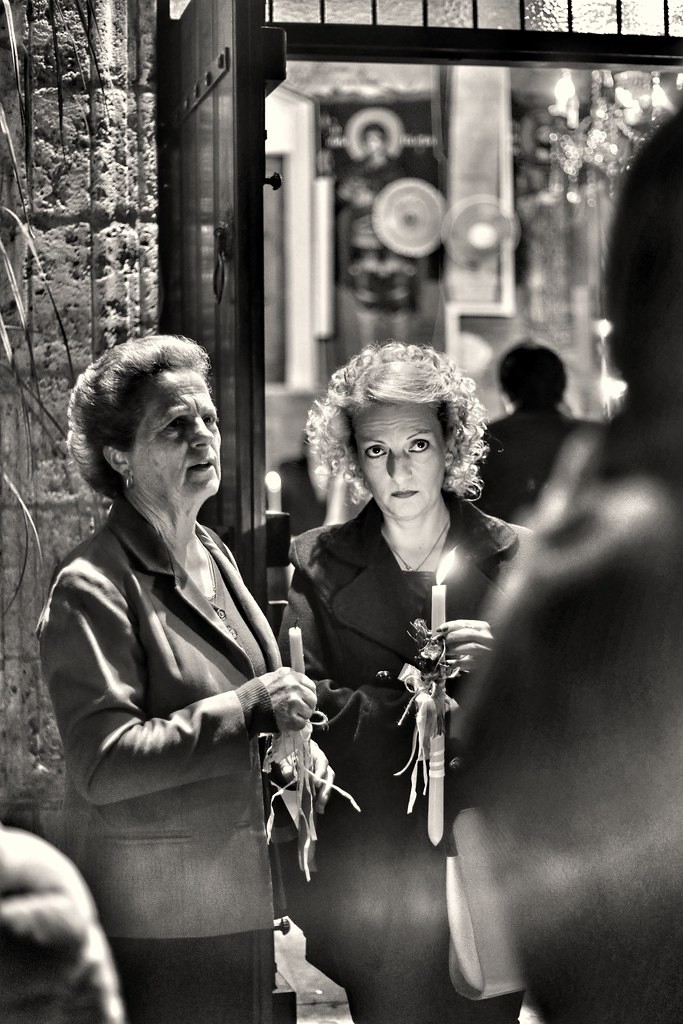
[
  {"left": 288, "top": 617, "right": 305, "bottom": 675},
  {"left": 431, "top": 545, "right": 459, "bottom": 638}
]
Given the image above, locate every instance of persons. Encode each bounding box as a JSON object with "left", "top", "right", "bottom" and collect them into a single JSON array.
[
  {"left": 36, "top": 331, "right": 318, "bottom": 1023},
  {"left": 477, "top": 103, "right": 683, "bottom": 1024},
  {"left": 287, "top": 340, "right": 534, "bottom": 1024}
]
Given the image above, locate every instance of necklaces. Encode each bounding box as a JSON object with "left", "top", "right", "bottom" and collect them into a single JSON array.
[{"left": 389, "top": 522, "right": 450, "bottom": 572}]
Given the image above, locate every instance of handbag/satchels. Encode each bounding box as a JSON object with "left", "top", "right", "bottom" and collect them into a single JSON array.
[{"left": 443, "top": 806, "right": 529, "bottom": 1002}]
